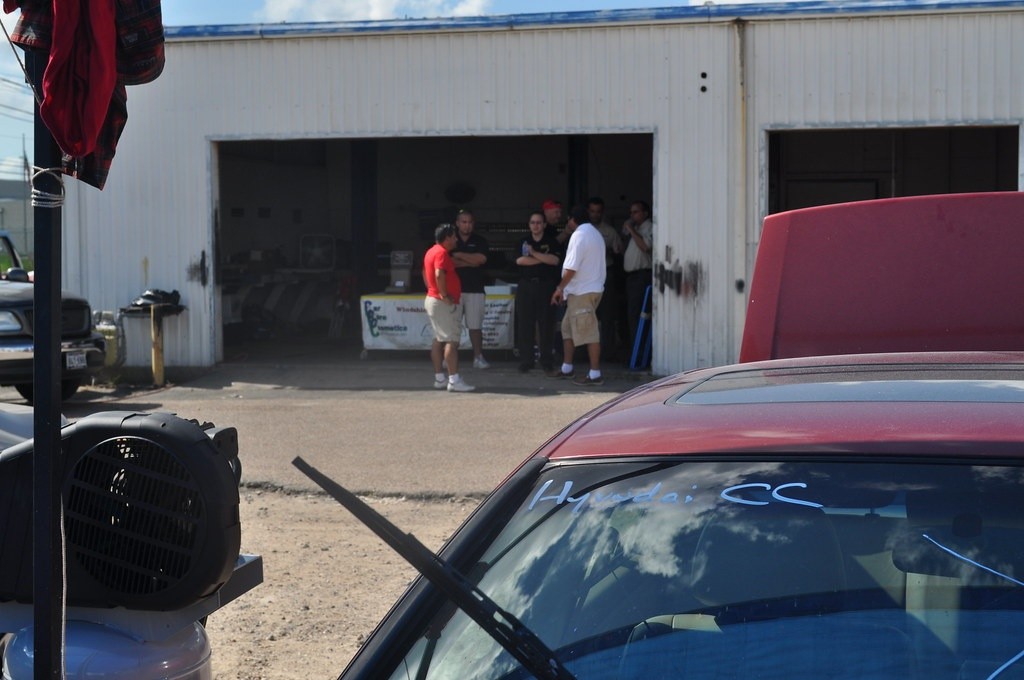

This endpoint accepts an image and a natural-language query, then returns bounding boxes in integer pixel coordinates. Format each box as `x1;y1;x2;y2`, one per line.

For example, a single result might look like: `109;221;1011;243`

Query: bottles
522;241;528;256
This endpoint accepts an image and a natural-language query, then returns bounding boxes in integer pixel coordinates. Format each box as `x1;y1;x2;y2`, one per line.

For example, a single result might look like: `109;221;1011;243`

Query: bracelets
556;286;563;292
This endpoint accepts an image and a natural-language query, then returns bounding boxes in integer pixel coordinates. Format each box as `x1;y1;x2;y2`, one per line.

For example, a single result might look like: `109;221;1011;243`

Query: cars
0;227;105;406
334;187;1024;680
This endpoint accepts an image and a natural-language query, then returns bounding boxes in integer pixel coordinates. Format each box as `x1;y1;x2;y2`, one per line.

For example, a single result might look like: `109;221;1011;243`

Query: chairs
798;459;905;629
618;503;915;680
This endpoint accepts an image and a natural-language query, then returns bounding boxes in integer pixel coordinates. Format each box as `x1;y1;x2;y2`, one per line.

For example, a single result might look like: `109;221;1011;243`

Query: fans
300;233;337;272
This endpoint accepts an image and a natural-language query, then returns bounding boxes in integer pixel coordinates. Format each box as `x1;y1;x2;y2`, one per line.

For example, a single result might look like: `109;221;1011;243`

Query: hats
544;200;560;211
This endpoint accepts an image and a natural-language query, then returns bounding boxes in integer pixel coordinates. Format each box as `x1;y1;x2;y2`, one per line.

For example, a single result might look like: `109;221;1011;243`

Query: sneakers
434;379;476;392
472;358;489;368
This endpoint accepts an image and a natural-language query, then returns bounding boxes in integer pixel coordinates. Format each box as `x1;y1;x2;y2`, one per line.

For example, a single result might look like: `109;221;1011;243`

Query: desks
360;292;514;360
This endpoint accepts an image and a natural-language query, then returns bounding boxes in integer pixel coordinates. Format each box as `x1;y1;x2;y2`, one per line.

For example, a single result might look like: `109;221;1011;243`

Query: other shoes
572;375;604;386
546;370;575;379
519;363;534;371
544;365;554;372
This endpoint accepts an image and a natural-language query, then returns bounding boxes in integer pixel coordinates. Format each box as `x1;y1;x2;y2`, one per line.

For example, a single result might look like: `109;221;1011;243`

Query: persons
546;210;606;385
541;198;577;253
512;212;560;370
422;223;475;392
587;198;622;345
623;202;652;363
442;210;489;370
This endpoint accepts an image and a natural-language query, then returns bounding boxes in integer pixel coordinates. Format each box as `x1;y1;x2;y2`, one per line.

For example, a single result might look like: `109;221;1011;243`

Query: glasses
530;221;542;225
445;222;450;234
630;210;639;214
